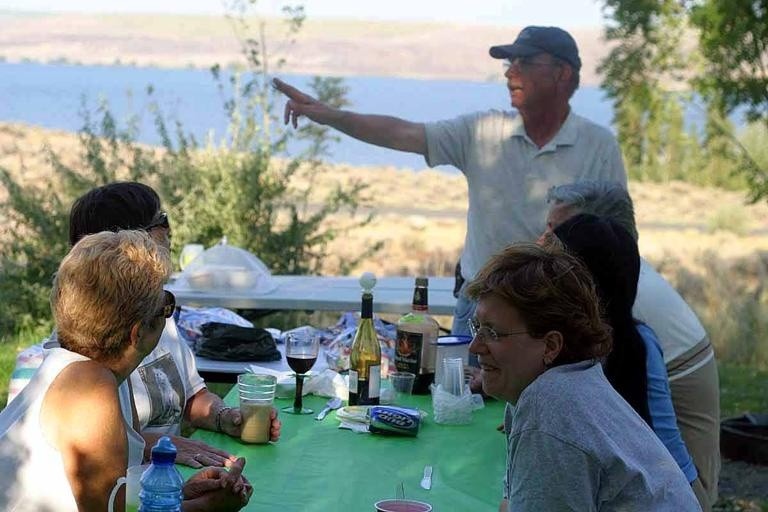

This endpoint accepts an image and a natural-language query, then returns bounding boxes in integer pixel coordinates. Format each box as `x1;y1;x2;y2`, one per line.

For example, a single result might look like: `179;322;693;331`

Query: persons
459;245;703;512
0;228;253;512
7;182;280;469
549;212;711;512
274;27;626;365
536;180;720;512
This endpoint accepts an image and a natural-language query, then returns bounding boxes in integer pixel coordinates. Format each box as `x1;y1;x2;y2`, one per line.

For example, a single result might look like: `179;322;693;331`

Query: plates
334;404;421;425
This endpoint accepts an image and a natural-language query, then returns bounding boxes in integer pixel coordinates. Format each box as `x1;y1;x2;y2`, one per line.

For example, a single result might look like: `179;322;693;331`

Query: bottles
348;292;381;406
395;275;437;395
139;435;185;512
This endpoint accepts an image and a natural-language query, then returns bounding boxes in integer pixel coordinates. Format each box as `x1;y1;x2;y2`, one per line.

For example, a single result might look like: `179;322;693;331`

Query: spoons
317;397;342;420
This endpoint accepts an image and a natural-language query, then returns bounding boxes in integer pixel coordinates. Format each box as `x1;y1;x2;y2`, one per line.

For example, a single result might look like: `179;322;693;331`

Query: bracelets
214;405;231;431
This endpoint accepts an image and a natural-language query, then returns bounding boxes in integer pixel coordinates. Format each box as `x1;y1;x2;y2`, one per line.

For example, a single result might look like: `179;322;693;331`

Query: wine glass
281;331;319;415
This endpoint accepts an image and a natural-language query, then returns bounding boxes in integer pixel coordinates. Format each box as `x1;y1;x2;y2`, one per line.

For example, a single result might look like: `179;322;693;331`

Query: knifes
419;466;434;490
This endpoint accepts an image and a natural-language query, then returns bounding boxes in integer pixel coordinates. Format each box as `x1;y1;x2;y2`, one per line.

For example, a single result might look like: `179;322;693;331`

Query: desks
196;339;327;384
162;275;459;316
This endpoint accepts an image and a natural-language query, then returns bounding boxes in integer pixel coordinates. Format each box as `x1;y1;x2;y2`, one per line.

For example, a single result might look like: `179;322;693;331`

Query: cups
107;465;149;512
390;372;416;406
435;357;466;423
176;243;206;275
233;374;277;446
374;499;433;512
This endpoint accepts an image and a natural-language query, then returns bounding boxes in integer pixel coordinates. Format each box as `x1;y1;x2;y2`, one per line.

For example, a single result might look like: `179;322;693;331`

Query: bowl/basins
186;273;215;291
223;269;260;291
720;417;767;462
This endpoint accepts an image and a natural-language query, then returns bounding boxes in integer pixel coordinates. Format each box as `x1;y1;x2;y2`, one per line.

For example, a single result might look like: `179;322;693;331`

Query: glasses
157;287;177;319
144;211;170;230
465;317;527;346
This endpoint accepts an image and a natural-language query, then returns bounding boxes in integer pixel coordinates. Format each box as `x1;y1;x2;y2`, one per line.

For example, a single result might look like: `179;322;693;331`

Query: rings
193;452;200;459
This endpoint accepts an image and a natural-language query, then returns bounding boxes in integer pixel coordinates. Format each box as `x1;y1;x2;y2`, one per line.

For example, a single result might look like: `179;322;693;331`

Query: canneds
366;406;422;437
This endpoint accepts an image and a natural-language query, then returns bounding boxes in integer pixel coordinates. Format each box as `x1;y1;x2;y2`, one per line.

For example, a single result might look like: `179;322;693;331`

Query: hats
488;24;583;69
